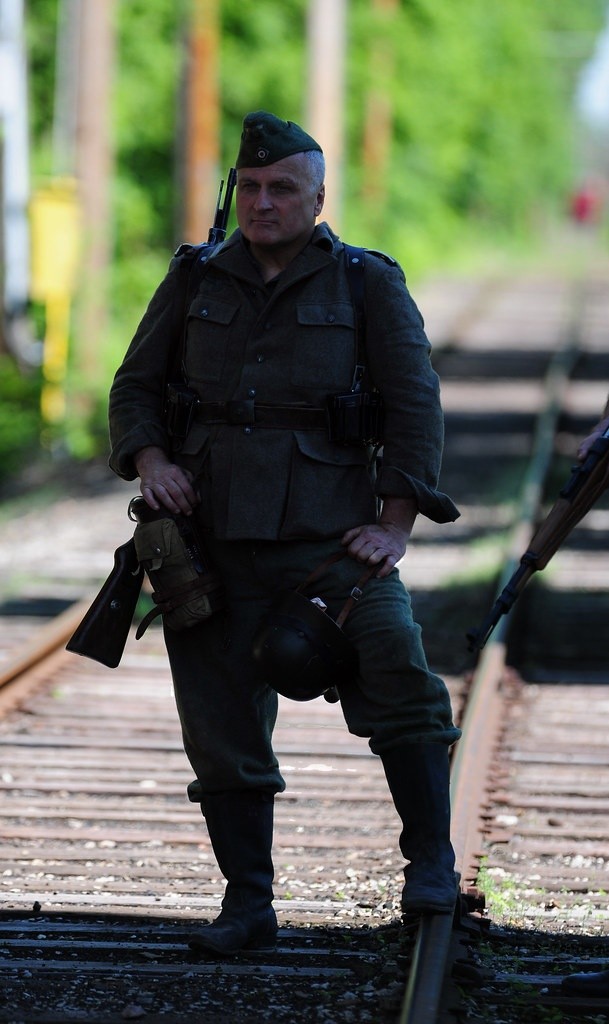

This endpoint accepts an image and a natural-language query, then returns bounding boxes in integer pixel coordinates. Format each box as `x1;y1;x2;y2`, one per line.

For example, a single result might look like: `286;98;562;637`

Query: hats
235;112;323;169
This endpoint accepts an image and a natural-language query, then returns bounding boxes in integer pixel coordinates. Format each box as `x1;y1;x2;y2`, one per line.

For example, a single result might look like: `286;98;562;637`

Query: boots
380;742;460;912
189;790;277;958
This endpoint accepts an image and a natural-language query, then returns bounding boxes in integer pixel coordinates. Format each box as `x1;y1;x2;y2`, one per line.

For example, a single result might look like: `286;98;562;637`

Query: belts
195;399;328;432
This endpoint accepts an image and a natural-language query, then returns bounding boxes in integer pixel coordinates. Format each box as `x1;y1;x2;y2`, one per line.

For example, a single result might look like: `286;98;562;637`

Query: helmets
252;589;357;700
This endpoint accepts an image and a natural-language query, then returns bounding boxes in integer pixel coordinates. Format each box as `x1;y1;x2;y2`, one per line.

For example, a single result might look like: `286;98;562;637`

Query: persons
107;111;461;961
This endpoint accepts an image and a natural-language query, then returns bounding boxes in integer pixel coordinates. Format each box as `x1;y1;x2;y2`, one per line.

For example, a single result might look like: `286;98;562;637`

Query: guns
465;398;609;653
67;166;239;668
